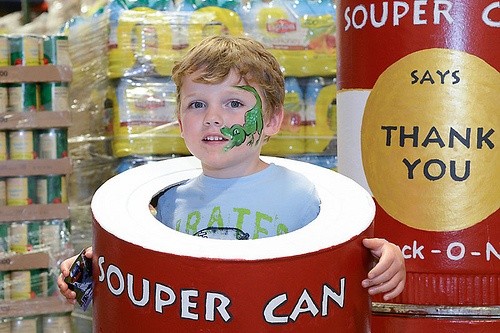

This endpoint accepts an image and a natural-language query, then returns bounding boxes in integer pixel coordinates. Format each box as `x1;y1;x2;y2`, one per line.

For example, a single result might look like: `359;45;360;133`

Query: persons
57;33;408;304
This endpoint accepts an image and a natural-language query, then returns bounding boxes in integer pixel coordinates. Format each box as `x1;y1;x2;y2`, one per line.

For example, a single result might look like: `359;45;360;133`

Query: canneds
0;32;71;333
